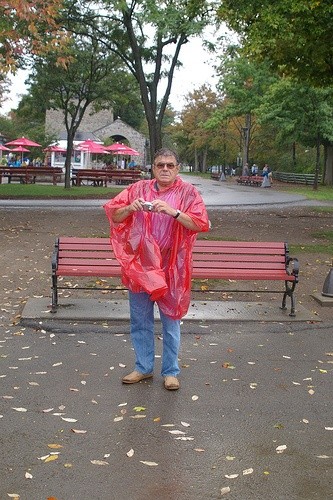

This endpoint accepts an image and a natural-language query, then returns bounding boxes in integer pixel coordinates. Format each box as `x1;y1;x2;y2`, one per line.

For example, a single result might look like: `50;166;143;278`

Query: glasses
153;161;178;169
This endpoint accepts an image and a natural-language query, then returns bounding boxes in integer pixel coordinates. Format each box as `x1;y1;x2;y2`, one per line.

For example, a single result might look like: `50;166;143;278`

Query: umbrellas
73;138;140;165
0;142;11;151
9;145;31;165
43;144;68;167
4;136;43;166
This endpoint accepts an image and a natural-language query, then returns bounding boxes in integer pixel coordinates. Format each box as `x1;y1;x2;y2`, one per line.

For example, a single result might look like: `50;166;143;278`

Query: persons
91;157;148;171
242;161;273;186
5;152;44;167
106;146;210;392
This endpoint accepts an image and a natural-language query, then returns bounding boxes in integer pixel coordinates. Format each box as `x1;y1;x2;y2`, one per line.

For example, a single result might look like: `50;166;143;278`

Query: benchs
0;164;143;186
210;173;265;187
48;233;300;316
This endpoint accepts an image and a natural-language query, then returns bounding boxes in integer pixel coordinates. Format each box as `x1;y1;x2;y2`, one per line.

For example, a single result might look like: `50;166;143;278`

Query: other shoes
164;375;180;389
122;370;153;383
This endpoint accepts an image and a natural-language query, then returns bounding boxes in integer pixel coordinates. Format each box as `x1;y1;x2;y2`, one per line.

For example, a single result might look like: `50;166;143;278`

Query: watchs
173;208;180;218
